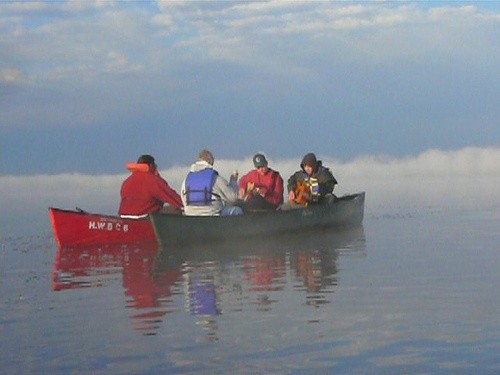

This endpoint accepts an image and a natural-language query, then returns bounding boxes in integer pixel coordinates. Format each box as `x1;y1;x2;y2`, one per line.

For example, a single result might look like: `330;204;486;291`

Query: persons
287;152;338;208
232;153;284;213
180;149;245;216
119;154;183;219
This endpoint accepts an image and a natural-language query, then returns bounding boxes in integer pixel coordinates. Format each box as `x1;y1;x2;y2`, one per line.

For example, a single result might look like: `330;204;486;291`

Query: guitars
289;179;311;205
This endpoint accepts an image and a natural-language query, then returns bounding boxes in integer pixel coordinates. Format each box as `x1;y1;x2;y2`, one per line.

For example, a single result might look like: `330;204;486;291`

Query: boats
149;190;367;247
51;243;159;294
47;205;156;247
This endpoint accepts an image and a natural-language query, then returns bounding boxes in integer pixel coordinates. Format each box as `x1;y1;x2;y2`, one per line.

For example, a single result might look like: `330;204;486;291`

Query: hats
253;153;268;167
